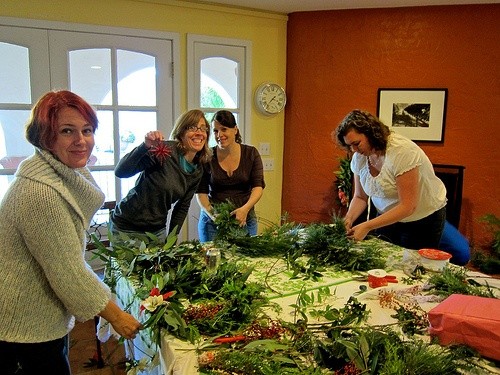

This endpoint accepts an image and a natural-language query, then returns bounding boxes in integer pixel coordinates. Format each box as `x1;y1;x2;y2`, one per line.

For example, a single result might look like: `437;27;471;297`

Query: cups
368;268;388;288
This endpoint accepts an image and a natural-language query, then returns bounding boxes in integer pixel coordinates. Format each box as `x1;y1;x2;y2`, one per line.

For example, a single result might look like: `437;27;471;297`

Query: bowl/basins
418;249;452;270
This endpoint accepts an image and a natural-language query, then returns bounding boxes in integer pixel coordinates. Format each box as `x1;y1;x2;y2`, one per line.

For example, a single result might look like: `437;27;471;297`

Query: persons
335;108;447;248
195;110;266;243
110;110;212;246
0;90;143;375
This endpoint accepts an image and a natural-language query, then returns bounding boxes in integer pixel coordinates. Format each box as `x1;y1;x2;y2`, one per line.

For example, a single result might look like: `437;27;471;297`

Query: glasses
185;125;209;132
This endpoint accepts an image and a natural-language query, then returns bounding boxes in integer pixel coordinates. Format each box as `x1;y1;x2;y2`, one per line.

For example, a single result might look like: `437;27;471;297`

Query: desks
96;223;500;375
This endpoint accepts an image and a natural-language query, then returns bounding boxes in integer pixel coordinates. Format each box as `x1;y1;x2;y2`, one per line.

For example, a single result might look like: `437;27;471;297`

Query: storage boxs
428;294;500;361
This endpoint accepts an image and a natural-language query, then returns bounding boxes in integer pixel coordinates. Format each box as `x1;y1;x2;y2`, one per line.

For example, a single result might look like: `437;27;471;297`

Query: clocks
255;82;287;116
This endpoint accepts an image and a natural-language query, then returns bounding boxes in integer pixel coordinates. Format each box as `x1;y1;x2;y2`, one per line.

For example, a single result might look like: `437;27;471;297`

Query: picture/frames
377;88;447;144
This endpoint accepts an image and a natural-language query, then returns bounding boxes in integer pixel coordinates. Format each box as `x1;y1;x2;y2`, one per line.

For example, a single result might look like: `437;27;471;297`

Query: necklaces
369;152;381;166
